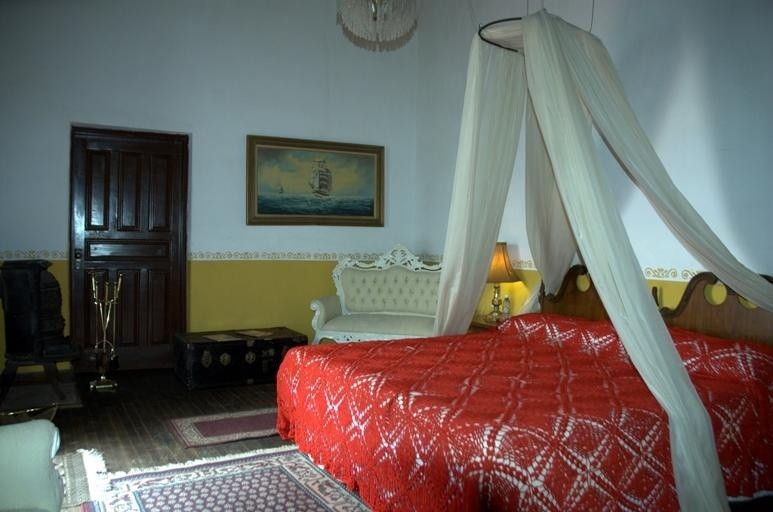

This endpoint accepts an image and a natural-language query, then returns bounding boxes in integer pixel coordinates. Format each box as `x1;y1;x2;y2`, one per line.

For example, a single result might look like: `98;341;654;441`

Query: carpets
58;449;108;508
81;444;373;512
167;406;278;449
0;380;83;409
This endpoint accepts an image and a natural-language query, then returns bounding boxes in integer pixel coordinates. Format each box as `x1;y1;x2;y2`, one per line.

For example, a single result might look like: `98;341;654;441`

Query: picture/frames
244;134;384;229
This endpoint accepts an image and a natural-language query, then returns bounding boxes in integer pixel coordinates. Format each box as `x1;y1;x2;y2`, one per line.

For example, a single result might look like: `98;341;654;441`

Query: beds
274;263;773;512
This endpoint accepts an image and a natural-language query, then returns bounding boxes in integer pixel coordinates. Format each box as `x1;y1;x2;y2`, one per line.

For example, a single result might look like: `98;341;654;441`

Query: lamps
334;0;420;56
483;241;520;323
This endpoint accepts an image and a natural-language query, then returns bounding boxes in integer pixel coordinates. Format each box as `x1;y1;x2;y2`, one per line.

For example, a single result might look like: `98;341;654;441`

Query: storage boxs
171;327;307;392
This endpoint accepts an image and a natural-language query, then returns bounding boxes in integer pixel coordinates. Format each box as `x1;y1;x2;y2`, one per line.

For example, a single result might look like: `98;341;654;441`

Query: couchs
308;243;443;345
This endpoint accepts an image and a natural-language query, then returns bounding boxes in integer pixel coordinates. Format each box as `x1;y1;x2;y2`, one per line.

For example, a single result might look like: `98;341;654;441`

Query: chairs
0;259;87;400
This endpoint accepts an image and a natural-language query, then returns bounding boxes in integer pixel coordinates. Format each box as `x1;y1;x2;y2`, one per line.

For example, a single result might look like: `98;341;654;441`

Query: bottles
501;293;511;313
